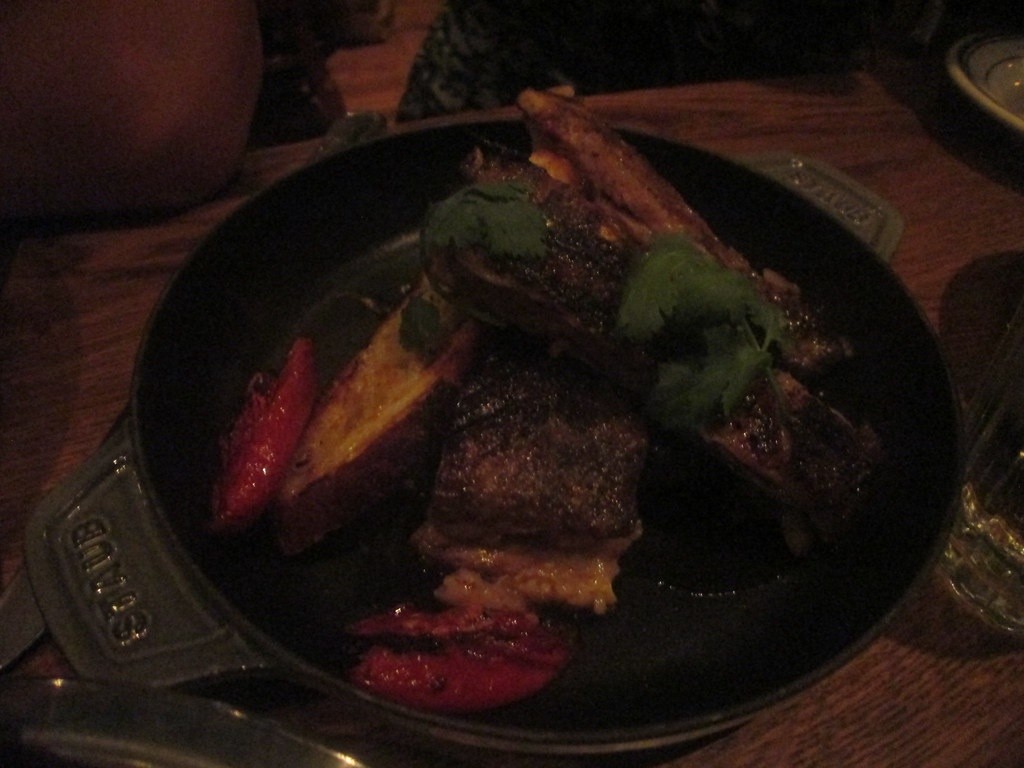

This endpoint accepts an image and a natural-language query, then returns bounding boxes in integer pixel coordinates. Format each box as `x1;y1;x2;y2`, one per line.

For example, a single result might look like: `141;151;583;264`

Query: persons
393;0;1024;125
0;0;267;218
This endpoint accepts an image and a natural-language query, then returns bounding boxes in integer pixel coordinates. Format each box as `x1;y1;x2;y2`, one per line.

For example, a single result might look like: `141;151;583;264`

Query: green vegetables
395;182;792;432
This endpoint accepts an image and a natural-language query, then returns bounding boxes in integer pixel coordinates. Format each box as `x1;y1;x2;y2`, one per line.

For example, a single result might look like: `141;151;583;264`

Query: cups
935;300;1024;638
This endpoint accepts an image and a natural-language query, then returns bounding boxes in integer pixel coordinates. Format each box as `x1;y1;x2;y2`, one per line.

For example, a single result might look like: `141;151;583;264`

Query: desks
0;46;1024;768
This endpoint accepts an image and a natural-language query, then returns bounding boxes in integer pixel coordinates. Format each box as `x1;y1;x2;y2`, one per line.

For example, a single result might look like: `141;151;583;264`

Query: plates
944;27;1024;136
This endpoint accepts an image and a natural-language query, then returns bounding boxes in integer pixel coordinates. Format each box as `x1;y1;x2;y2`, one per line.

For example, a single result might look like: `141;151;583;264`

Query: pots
23;115;968;768
